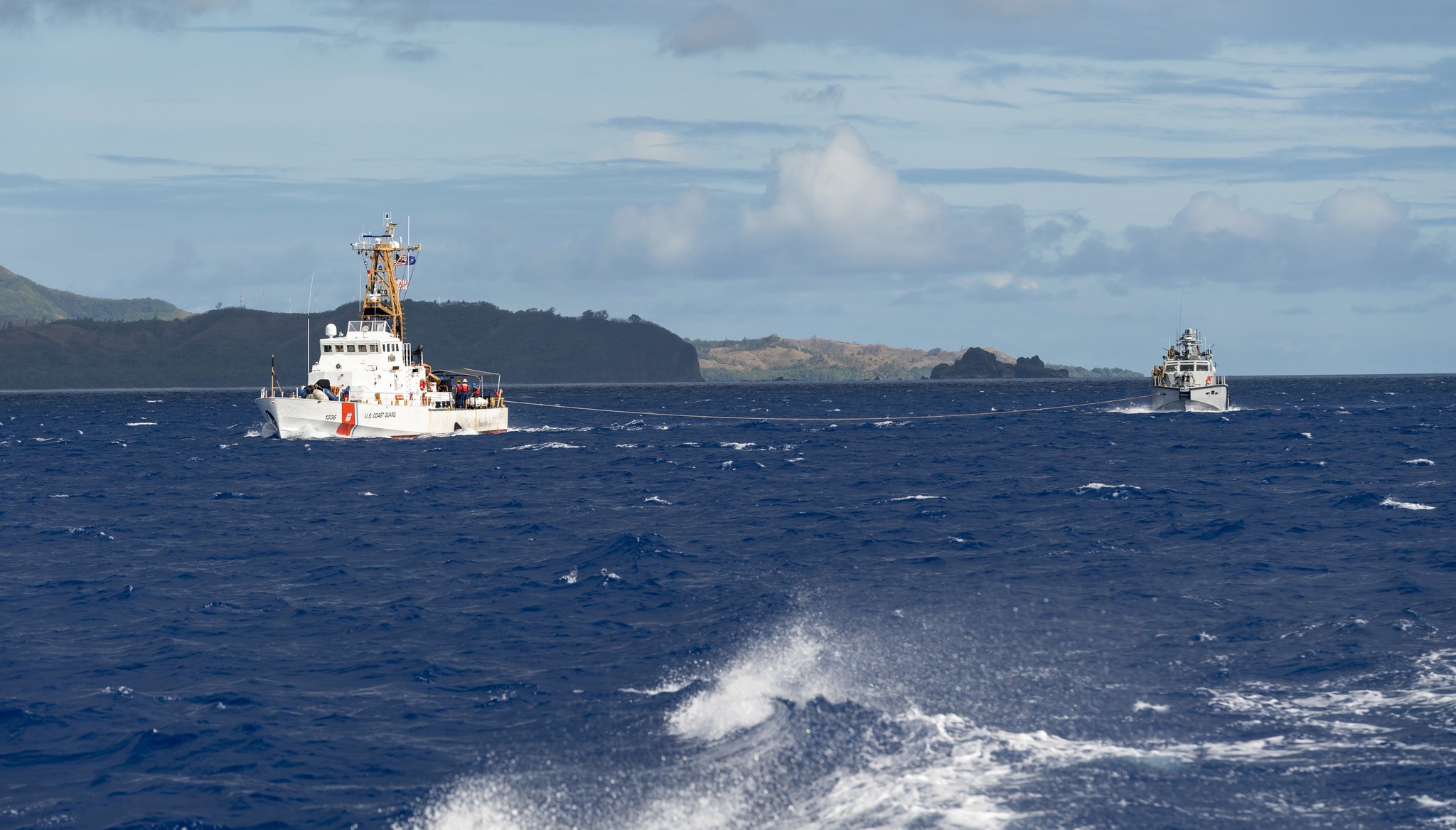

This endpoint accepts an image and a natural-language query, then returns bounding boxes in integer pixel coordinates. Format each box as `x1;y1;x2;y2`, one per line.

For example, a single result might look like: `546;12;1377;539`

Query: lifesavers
1206;376;1211;384
420;379;425;391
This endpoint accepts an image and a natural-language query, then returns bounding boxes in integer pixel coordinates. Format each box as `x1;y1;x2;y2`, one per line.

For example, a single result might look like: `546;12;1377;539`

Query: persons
1168;346;1180;360
1196;345;1205;356
436;376;445;409
460;379;470;407
363;317;372;332
470;383;480;398
446;379;454;407
1151;364;1165;386
456;379;462;408
381;316;393;334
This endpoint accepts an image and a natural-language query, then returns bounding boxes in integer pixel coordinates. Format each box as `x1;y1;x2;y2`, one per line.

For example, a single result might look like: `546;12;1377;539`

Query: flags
394;254;416;265
394;276;408;290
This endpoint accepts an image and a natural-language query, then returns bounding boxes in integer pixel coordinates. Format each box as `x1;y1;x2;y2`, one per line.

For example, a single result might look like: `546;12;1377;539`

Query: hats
387;316;391;319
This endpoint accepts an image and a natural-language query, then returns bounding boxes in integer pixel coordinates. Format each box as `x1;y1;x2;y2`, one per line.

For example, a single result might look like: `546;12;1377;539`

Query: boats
254;209;508;442
1148;276;1231;415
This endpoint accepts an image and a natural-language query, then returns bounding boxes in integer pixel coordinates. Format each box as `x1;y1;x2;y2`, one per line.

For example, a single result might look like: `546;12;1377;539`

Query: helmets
440;377;444;380
471;383;476;386
456;379;461;382
463;379;468;382
